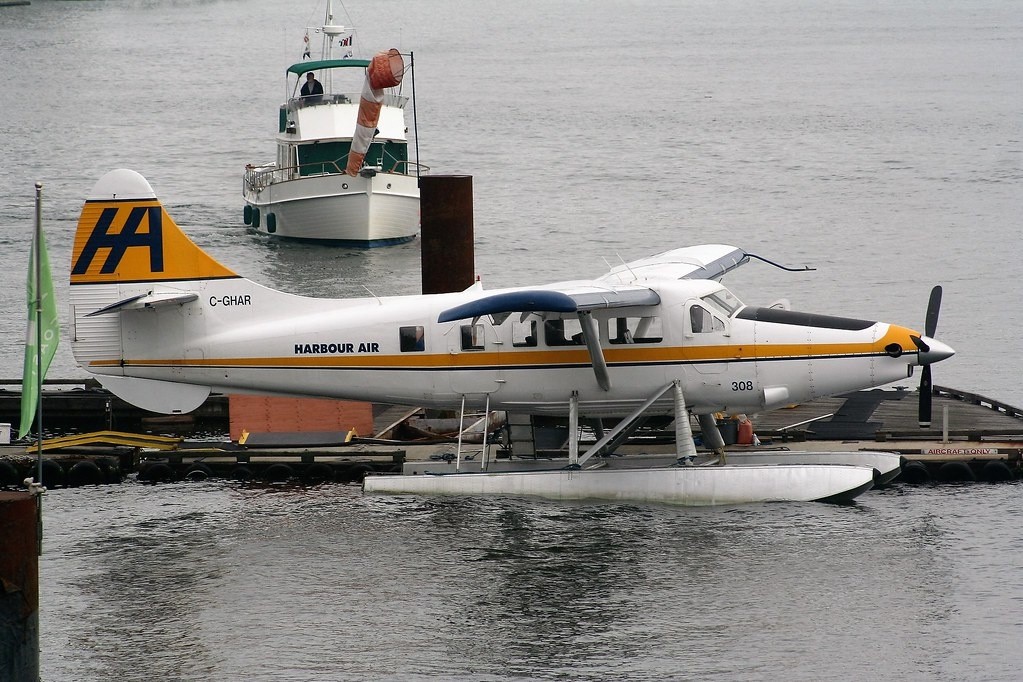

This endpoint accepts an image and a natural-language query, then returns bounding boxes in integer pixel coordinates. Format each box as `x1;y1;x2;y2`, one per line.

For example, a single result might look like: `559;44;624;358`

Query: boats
237;0;429;250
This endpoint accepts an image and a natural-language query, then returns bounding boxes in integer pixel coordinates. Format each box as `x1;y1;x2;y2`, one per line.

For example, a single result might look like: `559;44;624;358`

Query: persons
301;72;323;104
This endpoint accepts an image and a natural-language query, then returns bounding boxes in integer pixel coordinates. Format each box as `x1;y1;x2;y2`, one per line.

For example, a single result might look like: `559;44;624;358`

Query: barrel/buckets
0;423;11;444
716;419;737;444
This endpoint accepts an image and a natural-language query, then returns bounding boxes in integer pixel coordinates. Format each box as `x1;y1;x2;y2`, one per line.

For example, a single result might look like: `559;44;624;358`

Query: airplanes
61;165;960;505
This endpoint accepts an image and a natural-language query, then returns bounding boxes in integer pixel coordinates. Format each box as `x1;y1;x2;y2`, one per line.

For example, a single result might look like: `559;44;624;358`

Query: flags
18;225;59;440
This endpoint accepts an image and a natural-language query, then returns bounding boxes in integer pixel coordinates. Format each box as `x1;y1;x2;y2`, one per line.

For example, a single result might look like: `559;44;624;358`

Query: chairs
524;327;636;344
